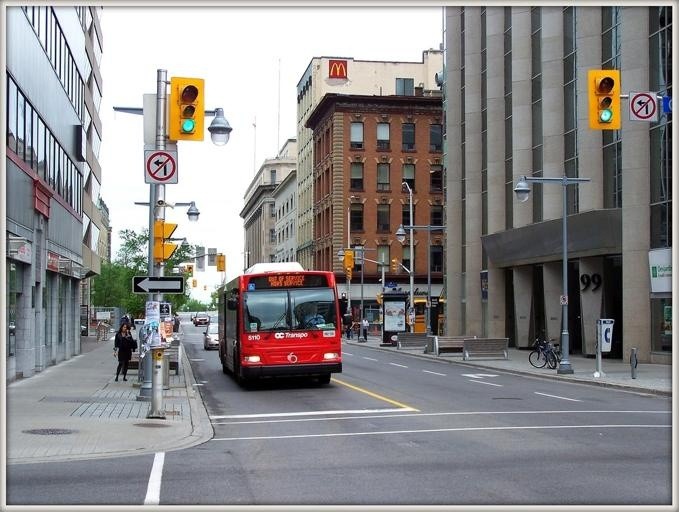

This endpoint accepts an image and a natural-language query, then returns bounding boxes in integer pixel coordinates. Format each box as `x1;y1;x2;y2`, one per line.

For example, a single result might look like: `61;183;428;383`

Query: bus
217;262;343;391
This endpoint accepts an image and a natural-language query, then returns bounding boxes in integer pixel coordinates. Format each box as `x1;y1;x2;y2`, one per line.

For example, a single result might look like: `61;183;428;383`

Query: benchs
127;347;178;374
397;332;508;360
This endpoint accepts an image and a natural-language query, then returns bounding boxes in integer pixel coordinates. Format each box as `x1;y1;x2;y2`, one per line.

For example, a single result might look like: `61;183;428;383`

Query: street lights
514;174;592;374
133;200;202;375
112;66;232;380
336;195;386;343
395;223;447;353
401;181;416;332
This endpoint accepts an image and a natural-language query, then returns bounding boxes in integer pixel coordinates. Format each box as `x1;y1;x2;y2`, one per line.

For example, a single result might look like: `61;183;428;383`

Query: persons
363;318;369;341
113;315;137;382
346;318;359;339
305;304;325;328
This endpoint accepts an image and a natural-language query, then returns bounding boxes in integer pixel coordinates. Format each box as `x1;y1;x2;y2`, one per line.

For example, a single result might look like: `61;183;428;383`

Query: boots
115;377;128;381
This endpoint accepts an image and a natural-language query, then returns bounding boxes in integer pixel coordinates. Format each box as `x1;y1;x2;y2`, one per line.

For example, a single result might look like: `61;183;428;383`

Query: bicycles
528;333;562;369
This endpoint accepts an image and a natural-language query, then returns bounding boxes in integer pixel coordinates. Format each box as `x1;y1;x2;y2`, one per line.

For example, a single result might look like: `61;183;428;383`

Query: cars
190;312;219;351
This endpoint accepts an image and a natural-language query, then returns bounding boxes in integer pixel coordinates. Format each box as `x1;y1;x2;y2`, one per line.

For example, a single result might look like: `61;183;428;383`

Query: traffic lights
170;76;204;142
587;69;622;129
187;265;193;277
